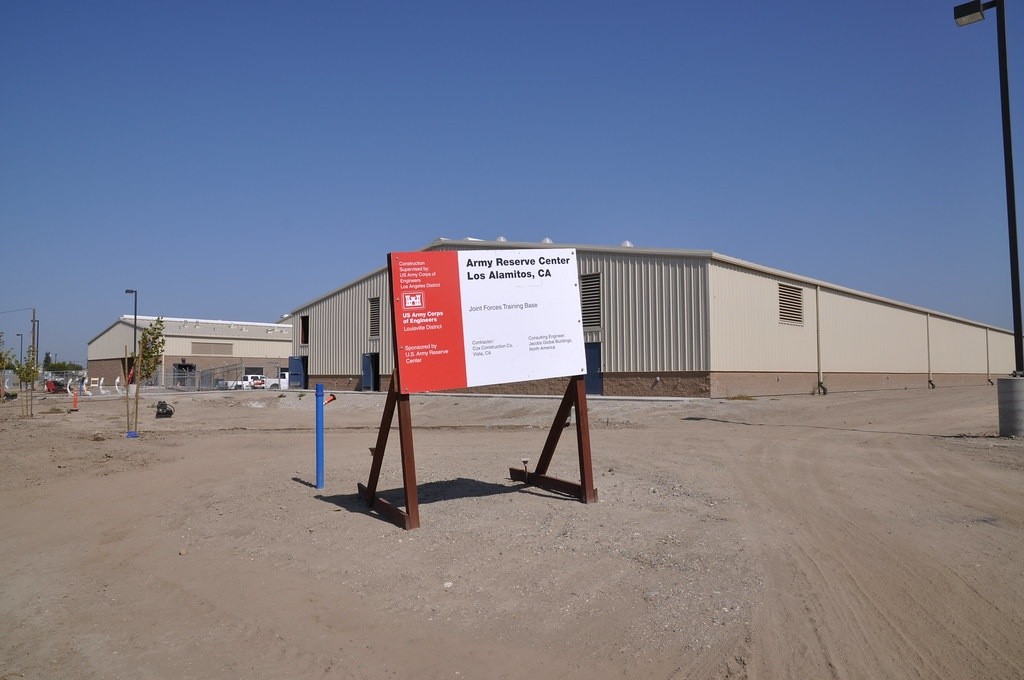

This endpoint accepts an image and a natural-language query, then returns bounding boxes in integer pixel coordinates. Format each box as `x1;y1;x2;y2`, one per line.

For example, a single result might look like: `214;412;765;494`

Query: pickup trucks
218;374;266;390
252;371;289;390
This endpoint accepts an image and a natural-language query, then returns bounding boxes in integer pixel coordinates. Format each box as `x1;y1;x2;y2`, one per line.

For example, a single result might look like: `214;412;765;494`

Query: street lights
55;353;58;364
16;333;23;366
31;319;39;372
126;289;139;387
952;1;1024;453
